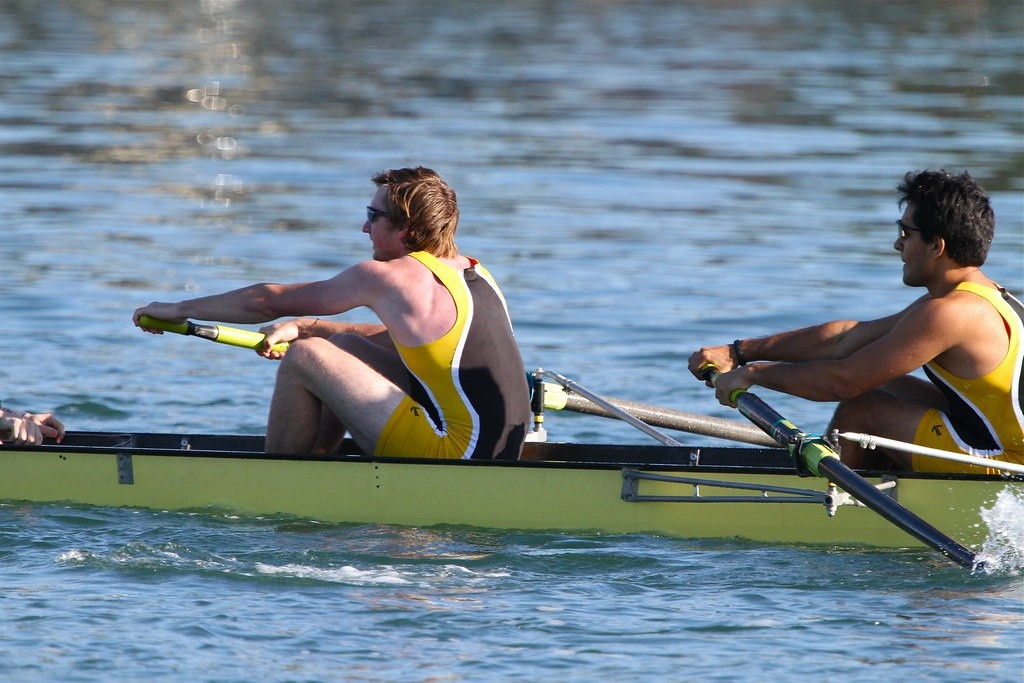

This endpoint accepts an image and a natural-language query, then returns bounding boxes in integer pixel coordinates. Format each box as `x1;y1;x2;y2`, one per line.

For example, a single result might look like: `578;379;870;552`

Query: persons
688;175;1024;475
0;406;65;445
132;166;531;460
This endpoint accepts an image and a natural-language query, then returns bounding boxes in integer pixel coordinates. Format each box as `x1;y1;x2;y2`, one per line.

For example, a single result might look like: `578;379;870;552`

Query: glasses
367;206;397;222
896;219;926;239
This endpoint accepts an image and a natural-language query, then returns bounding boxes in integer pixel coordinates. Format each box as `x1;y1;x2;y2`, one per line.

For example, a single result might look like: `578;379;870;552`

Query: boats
1;367;1024;553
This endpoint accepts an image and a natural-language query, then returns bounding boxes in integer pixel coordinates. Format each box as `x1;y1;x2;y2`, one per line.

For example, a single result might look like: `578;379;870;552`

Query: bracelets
734;340;746;366
